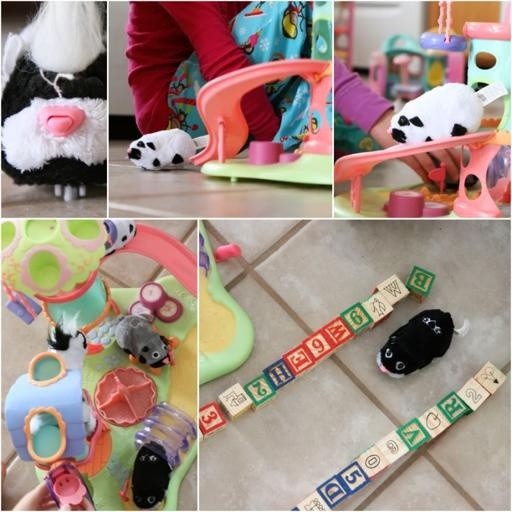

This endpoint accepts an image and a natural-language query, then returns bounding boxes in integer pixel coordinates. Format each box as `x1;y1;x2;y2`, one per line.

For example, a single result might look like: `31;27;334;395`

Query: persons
2;462;60;510
126;1;333;155
334;52;400;148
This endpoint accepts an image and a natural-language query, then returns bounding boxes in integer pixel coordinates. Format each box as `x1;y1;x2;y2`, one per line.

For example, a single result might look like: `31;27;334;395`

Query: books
376;308;471;379
